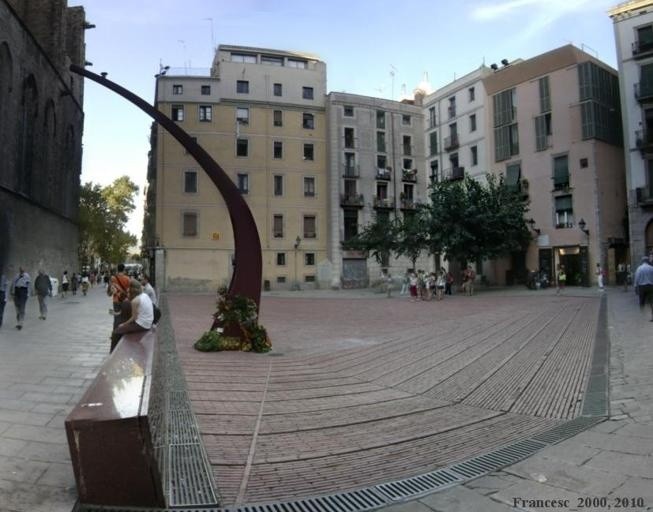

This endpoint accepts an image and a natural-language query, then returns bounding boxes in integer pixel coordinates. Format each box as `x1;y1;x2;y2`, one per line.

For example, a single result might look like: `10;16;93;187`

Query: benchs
62;330;167;508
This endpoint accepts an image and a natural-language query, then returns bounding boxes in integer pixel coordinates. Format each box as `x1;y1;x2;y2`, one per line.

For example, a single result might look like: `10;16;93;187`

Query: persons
0;264;157;354
387;256;653;310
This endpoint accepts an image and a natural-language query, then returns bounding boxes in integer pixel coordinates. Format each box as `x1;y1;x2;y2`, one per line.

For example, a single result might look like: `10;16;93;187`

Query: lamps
578;216;589;237
527;216;540;235
